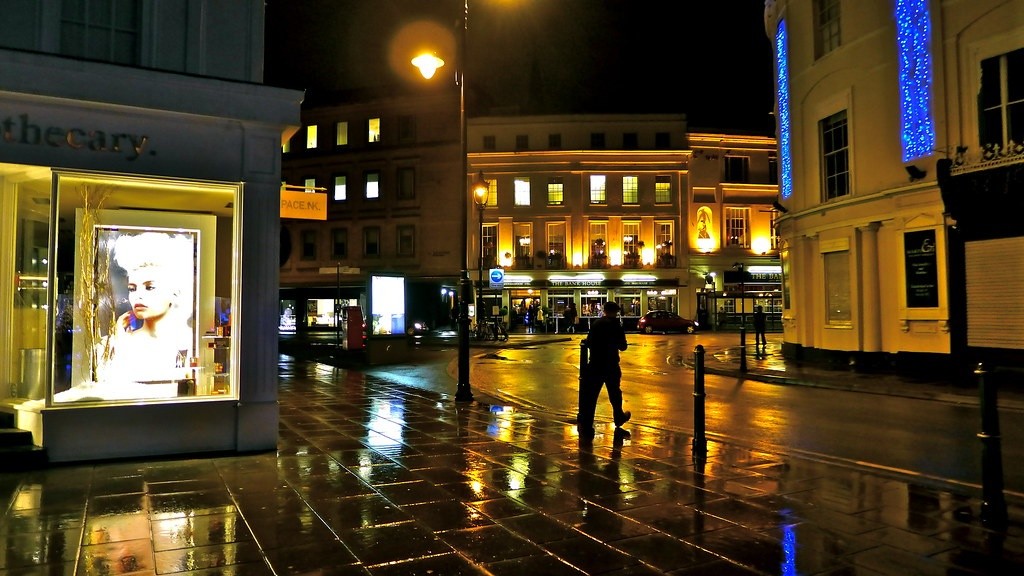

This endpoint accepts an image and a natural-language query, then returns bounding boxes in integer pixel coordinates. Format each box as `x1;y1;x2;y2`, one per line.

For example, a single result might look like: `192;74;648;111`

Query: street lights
409;28;474;403
710;270;720;331
472;170;491;321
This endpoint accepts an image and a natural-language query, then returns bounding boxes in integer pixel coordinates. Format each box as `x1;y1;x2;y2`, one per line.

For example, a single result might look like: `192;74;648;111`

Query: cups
189;358;197;367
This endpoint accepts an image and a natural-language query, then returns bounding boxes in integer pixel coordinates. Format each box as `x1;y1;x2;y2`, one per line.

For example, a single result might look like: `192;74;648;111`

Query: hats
604;301;621;312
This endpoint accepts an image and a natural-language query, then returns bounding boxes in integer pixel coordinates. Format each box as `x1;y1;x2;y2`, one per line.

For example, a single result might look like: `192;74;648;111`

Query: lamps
773;202;788;214
505;253;512;259
762;252;766;256
706;252;711;256
905;165;927;182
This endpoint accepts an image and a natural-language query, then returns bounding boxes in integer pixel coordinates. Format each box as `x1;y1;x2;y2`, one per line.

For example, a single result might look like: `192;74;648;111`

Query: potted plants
500;307;508;333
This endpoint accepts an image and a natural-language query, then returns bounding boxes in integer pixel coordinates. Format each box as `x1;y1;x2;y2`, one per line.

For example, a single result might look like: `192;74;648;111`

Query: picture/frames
71;206;218;400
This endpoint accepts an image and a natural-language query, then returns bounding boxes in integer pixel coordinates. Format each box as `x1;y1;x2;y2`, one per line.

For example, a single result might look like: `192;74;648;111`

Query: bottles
175;351;185;368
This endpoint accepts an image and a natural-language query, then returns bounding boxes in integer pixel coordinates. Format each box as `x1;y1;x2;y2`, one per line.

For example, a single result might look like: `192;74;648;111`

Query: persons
564;301;577;334
753;306;767;350
97;232;195;379
528;305;535;333
718;307;727;325
582;302;632;427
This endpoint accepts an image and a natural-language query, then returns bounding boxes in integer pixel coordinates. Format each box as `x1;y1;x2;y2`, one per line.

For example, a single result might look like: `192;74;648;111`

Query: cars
637;310;700;334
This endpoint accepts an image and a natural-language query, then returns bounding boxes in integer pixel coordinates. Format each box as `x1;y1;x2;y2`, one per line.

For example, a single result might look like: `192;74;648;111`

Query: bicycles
470;317;511;341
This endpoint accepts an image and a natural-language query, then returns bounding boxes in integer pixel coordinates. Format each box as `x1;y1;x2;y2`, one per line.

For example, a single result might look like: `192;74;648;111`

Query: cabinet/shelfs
202;334;233;396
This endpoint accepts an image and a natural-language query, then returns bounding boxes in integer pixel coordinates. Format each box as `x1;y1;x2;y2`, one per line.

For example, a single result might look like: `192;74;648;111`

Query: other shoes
613;411;631;426
